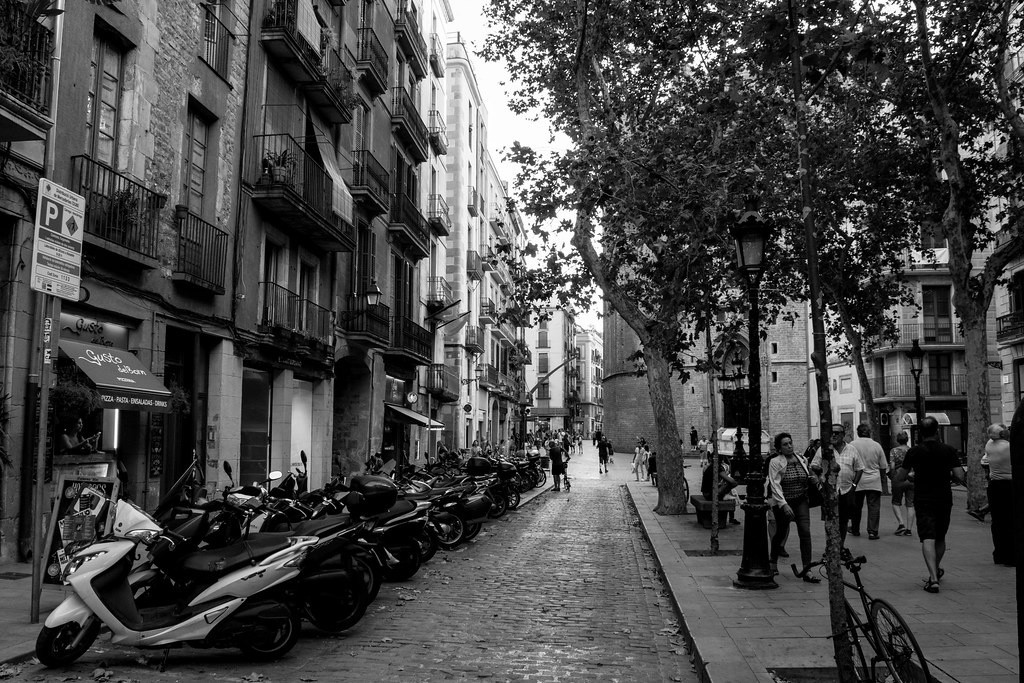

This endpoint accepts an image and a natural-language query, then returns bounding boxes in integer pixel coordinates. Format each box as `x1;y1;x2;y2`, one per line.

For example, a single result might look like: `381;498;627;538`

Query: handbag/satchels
806;483;825;508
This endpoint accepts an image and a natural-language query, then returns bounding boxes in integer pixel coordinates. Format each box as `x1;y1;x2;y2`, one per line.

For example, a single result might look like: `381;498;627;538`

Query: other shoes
779;546;789;558
729;518;741;525
904;530;912;536
968;508;984;521
770;563;779;575
895;524;905;533
803;574;821;582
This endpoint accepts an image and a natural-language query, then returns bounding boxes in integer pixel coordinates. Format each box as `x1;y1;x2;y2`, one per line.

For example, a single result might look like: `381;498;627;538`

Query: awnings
901;412;961;431
384;404;445;431
58;339;172;413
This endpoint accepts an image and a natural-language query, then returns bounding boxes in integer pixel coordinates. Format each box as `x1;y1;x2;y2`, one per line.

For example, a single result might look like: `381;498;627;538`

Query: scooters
155;439;539;581
35;488;319;666
130;503;372;634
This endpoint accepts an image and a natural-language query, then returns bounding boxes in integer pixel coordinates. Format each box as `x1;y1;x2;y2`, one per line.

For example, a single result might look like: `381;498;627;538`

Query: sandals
847;526;861;537
868;531;880;540
924;568;944;593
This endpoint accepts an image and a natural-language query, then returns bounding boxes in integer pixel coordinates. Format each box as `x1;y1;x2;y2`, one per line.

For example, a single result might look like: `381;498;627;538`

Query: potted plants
168;380;191;415
254;320;335;354
49;381;103;415
265;148;300;182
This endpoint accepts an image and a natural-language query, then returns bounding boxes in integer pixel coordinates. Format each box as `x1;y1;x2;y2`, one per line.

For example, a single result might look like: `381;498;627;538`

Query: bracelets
852;483;857;487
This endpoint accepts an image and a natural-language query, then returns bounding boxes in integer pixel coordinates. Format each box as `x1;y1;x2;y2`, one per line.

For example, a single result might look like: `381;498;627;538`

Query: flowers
89;183;153;228
152;172;171;194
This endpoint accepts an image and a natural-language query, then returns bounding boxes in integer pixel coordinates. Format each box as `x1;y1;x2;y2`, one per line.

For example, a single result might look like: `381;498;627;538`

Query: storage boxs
467;457;491;475
527;449;540;462
350;475;397;517
497;462;517;481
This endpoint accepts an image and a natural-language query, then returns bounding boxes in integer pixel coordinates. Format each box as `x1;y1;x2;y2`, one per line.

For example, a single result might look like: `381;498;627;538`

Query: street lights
904;338;926;444
729;353;747;458
730;210;776;584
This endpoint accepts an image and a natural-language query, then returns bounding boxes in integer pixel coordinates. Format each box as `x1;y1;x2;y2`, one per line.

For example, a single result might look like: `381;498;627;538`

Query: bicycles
563;457;572;492
510;452;546;488
791;542;931;683
684;464;690;501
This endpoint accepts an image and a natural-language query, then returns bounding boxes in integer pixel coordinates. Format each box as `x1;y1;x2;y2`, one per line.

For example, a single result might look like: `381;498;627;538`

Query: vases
174;205;189;219
105;226;125;243
147;194;168;208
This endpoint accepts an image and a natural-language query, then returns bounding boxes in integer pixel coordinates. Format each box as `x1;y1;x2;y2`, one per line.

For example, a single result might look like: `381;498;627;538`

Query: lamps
340;277;383;325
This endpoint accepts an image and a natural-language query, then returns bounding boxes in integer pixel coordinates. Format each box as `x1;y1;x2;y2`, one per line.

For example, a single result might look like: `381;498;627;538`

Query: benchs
691;496;736;529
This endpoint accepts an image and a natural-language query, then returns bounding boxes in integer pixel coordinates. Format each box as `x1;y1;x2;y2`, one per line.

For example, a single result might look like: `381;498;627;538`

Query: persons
528;429;583;457
980;422;1015;567
897;416;965;593
437;441;448;461
762;451;789;558
471;436;491;457
803;438;821;464
633;437;656;481
593;429;614;474
61;415;102;452
690;426;741;525
847;423;889;540
889;432;914;535
768;433;822;584
548;441;571;491
810;424;865;548
